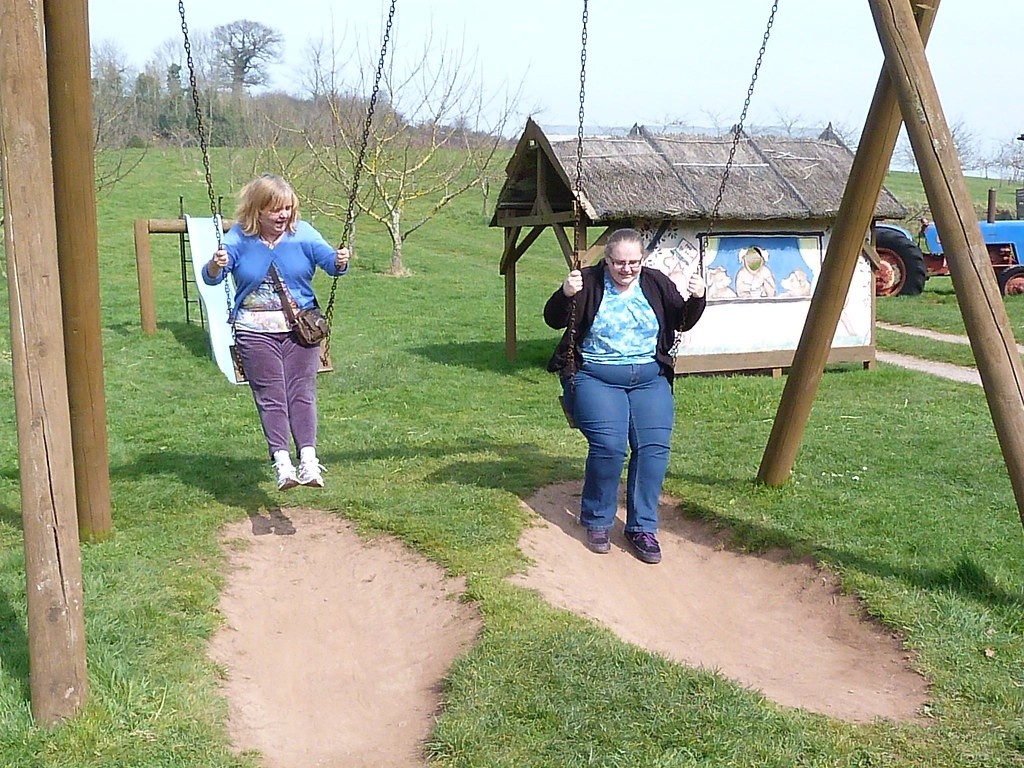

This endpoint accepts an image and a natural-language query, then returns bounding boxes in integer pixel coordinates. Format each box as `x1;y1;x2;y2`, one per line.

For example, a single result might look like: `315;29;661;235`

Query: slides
181;214;249;387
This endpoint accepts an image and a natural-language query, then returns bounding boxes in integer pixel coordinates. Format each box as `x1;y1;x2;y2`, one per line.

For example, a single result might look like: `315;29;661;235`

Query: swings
168;2;399;386
559;0;781;429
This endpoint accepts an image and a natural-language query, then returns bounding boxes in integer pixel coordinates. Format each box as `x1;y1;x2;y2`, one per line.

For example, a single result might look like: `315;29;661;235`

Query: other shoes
624;524;662;563
588;528;611;553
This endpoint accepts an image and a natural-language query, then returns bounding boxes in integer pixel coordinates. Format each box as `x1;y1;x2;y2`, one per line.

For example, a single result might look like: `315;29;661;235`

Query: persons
544;228;707;565
202;174;350;490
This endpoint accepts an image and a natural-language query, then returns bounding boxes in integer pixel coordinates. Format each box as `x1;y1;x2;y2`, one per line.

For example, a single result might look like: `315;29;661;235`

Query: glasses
608;255;643;268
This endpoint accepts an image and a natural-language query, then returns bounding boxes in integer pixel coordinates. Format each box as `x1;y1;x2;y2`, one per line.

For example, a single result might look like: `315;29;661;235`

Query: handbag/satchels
291;307;329;347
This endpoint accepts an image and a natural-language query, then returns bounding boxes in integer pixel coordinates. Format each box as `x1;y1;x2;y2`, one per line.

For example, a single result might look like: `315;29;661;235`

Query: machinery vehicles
872;187;1024;302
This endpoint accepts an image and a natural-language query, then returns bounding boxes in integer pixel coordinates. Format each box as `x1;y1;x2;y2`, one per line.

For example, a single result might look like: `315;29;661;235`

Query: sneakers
299;457;327;487
272;462;301;491
